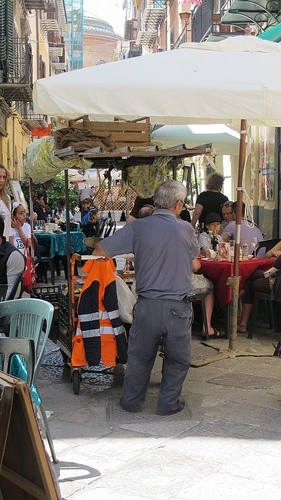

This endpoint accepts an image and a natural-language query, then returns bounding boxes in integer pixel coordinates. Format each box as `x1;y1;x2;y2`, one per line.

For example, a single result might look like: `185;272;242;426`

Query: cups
116;258;126;269
206;249;213;260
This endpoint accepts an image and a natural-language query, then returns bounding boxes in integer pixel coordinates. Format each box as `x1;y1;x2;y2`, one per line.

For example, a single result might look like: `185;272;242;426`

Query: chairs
0;298;58;464
190;239;281;340
96;217;116;239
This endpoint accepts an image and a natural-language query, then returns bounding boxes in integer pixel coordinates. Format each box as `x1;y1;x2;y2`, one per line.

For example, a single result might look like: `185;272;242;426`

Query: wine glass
250;237;260;260
34;220;44;230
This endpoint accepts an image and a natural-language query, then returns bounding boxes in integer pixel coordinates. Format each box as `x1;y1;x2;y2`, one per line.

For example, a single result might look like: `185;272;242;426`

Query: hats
205;213;222;225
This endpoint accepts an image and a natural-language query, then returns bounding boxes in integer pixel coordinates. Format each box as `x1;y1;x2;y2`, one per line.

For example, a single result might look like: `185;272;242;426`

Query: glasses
177;199;187;210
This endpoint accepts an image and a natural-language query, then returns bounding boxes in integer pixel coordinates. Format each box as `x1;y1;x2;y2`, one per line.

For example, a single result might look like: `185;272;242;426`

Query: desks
34;232;84;285
197;255;272;305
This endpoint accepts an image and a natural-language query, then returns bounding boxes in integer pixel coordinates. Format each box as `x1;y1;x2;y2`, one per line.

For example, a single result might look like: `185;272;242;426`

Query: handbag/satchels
21;257;38;295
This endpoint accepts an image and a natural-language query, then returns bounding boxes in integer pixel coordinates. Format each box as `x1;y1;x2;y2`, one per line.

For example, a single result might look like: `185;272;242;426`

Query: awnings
220;0;281;34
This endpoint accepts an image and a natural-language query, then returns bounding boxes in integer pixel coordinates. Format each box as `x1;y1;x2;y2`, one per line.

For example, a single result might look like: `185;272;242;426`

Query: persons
237;241;281;332
179;201;264;260
189;173;228;229
80;198;98;238
52;198;74;223
138;204;226;339
33;193;52;223
90;180;200;414
0;164;37;302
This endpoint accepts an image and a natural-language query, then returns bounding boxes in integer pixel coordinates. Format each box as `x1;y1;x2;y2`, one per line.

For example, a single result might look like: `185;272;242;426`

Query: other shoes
155;398;185;415
119;396;144;412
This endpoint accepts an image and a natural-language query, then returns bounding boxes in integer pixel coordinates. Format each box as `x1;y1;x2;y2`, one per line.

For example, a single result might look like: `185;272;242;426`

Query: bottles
45;214;58;234
211;230;248;262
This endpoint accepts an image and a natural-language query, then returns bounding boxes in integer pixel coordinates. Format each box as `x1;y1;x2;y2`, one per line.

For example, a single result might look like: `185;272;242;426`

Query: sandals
202;328;226;338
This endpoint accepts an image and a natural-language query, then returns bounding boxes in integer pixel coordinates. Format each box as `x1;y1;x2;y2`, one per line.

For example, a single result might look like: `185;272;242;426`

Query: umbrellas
31;35;281;127
150;124;247;156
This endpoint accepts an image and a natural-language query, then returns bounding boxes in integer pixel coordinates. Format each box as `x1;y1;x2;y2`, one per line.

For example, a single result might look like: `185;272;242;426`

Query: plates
33;230;44;233
